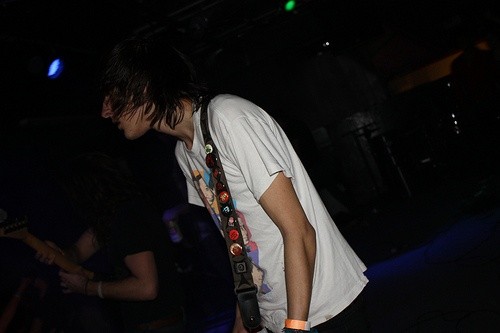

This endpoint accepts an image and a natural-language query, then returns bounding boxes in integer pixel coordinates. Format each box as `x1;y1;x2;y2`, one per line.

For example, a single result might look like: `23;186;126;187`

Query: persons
295;38;500;218
35;167;185;333
101;30;377;333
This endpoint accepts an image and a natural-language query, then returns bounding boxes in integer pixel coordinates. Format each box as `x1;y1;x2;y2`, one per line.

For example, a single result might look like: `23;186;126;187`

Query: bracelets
97;281;107;299
81;278;89;297
281;318;311;333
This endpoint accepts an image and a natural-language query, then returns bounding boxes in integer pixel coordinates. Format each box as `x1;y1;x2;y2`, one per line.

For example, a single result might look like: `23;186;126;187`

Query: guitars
0;213;95;281
